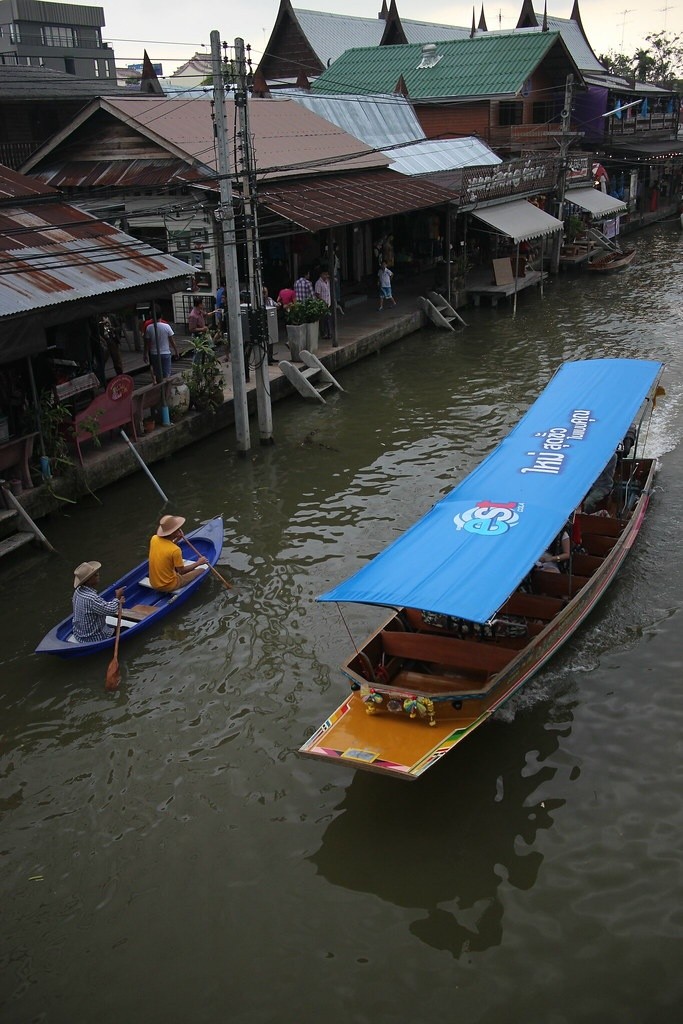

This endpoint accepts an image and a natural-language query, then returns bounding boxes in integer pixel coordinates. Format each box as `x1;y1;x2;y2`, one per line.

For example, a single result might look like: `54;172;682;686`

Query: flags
641;99;647;117
615;100;621;119
666;99;672;112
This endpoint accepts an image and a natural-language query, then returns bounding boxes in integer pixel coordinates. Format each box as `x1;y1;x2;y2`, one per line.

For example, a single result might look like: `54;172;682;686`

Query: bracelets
557;556;560;562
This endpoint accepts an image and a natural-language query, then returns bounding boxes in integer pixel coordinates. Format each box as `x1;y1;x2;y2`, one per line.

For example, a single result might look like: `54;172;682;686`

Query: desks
561;241;597;257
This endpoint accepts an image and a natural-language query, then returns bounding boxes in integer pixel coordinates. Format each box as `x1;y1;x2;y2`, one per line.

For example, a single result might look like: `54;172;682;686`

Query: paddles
181;535;233;590
105;601;123;690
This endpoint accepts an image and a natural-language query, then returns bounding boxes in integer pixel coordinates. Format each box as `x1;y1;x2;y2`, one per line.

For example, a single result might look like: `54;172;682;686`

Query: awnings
564;186;627;262
471;199;564;314
611;140;683;220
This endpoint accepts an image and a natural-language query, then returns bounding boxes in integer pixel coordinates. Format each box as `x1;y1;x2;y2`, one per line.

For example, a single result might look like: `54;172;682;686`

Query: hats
157;515;186;537
74;560;102;588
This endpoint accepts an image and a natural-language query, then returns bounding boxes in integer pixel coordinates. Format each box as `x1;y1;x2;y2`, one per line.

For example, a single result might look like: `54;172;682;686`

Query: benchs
129;376;175;438
139;577;184;593
0;431;38;490
73;374;137;467
380;490;636;679
105;608;145;630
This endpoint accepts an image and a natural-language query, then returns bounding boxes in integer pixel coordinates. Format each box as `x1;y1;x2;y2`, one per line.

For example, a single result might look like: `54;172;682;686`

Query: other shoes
391;303;397;307
377;307;384;311
270;359;279;363
322;335;331;338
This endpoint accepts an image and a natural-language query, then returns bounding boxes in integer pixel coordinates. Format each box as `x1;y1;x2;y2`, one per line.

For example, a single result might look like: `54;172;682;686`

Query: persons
72;562;129;643
213;284;226;323
543;525;570;572
143;303;179;385
104;312;122;376
378;260;396;311
149;515;209;592
189;298;221;366
584;452;617;514
315;269;332;339
293;269;317;303
262;281;296;362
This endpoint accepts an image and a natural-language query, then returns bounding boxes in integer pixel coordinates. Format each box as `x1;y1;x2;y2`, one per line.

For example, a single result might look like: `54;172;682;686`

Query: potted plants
180;332;233;416
280;296;330;363
23;391;74;480
449;251;475;292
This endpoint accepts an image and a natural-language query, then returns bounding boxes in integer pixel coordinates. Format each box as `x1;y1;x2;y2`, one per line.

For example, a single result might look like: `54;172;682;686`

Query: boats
31;509;224;665
581;249;638;272
297;358;663;781
